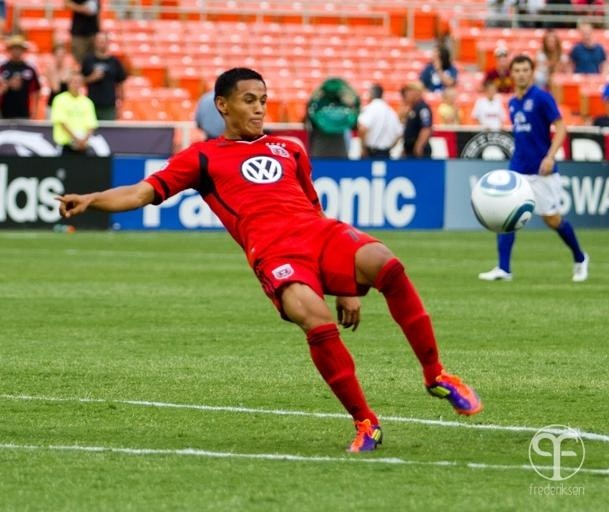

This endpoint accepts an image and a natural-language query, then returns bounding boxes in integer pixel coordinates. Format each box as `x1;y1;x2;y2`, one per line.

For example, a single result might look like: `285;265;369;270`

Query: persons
56;66;482;455
0;1;608;160
477;55;589;284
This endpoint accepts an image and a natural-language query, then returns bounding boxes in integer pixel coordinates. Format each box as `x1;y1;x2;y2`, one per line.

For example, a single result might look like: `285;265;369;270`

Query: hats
6;36;28;52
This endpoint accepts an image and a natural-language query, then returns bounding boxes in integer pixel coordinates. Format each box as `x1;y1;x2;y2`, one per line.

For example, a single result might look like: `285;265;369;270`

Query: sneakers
572;252;589;282
479;266;514;281
348;419;382;452
425;368;484;417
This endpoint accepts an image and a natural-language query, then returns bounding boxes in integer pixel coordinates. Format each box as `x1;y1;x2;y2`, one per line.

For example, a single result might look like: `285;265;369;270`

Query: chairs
0;0;608;127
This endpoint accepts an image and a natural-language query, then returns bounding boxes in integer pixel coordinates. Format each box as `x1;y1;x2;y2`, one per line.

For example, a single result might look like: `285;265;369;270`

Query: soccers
471;170;535;233
